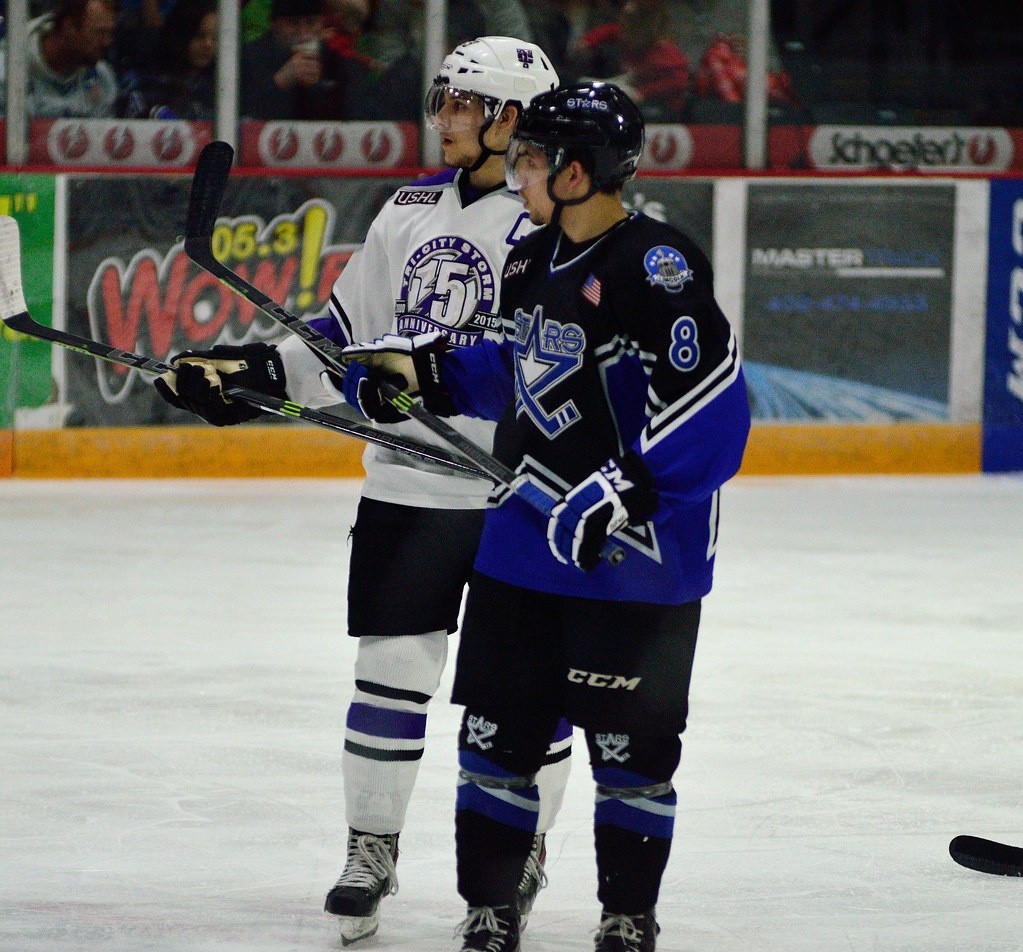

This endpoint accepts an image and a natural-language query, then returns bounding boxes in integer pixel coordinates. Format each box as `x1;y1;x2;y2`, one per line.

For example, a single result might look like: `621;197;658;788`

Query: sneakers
452;904;520;952
514;833;547;931
323;828;402;948
588;909;660;952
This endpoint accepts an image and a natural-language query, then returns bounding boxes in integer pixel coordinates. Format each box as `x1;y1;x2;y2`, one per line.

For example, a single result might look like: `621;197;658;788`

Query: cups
289;37;321;85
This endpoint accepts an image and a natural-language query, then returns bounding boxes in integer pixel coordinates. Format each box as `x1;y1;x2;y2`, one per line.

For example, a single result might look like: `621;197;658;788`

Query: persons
154;35;560;944
0;0;816;127
320;80;752;952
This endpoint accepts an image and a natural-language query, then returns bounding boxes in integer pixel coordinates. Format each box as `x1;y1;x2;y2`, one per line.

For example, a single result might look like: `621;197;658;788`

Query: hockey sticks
181;139;628;565
948;835;1023;877
0;212;497;486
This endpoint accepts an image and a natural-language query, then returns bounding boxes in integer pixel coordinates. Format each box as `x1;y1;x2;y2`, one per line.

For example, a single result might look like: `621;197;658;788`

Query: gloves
155;342;293;427
344;330;444;355
546;450;660;575
326;338;464;425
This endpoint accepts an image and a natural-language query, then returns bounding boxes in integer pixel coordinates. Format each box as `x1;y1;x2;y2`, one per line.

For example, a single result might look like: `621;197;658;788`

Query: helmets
432;36;559;122
514;80;645;187
